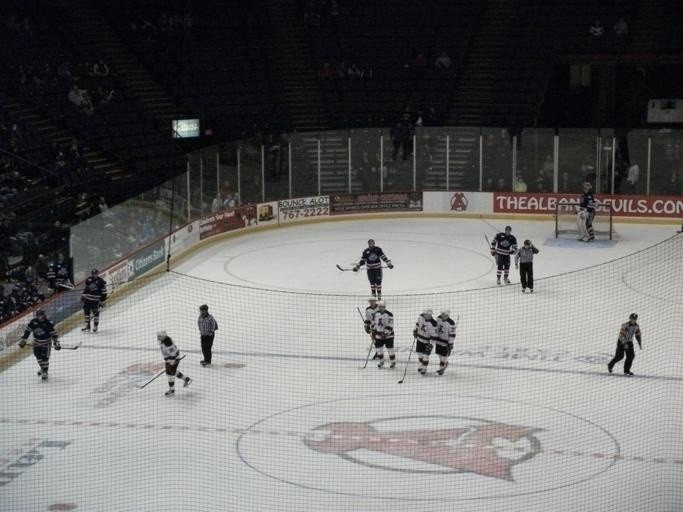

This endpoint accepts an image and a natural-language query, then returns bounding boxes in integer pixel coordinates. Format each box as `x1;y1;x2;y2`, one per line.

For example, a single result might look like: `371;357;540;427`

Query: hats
368;296;450;318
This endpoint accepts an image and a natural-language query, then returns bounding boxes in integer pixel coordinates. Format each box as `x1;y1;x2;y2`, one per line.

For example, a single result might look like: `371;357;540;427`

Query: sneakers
608;363;613;372
624;371;633;375
82;325;90;331
93;326;98;331
37;369;48;379
497;278;533;294
378;361;449;375
164;361;211;395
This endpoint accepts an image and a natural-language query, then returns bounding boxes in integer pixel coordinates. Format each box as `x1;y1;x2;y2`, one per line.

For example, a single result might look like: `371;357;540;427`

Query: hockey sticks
398;334;417;383
25;341;82;350
438;315;459;372
337;265;390;271
133;355;184;389
356;332;374;369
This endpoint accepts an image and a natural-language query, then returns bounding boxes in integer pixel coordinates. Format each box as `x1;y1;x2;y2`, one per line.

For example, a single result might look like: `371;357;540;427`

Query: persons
413;52;451;71
608;313;643;375
80;268;107;332
354;106;439;190
484;133;680;196
363;298;456;374
319;60;374;81
211;121;289;213
491;226;539;293
19;310;60;380
156;331;191;396
304;2;339;27
579;183;596;242
590;18;629;37
0;2;195;321
197;305;216;366
354;239;394;302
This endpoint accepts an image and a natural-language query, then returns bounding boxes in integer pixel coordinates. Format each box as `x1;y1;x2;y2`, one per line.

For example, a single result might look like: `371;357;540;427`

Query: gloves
509;250;514;255
81;294;86;302
100;301;106;307
53;341;61;351
491;252;496;256
353;267;360;272
388;263;393;269
18;340;27;348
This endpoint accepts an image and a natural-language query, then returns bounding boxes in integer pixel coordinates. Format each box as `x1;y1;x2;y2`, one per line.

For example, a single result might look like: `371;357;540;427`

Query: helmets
524;240;531;245
36;309;45;317
199;305;209;310
505;226;512;231
92;269;98;274
629;313;638;320
368;239;375;244
156;330;167;336
